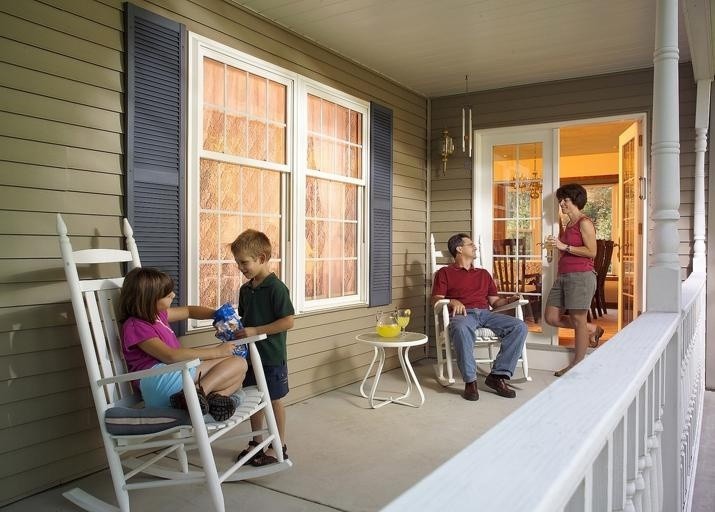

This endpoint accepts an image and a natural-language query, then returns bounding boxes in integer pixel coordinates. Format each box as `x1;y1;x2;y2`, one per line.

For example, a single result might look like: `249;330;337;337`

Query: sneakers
206;392;236;421
170;371;209;415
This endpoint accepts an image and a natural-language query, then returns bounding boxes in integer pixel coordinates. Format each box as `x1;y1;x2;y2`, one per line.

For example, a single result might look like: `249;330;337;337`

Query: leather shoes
485;374;516;398
464;382;479;401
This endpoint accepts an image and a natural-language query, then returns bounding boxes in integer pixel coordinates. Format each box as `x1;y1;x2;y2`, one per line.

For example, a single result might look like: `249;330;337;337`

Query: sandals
238;441;263;466
589;326;604;348
555;369;566;377
252;444;288;467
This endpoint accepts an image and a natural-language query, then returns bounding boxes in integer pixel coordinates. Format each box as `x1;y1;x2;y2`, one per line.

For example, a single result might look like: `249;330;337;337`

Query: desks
356;332;428;411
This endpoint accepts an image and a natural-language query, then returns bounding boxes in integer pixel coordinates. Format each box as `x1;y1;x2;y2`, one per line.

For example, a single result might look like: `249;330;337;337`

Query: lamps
440;128;454;175
512;144;542;200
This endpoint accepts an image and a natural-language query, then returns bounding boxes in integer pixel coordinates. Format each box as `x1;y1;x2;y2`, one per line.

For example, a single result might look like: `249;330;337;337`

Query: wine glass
543;235;556;257
398;309;411;337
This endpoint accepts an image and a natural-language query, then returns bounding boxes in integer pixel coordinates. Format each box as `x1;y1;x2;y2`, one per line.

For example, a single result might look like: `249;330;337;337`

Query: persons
116;265;250;421
544;182;605;377
227;228;296;467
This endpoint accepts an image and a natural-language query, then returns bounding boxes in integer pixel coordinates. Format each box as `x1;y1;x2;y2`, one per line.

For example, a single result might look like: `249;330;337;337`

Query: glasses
460;243;476;248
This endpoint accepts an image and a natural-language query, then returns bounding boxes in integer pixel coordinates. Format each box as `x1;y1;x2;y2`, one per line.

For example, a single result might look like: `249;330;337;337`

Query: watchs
564;244;570;253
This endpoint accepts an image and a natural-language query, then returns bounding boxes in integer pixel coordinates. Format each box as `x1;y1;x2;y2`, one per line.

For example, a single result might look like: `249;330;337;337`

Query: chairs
57;213;294;512
584;240;614;322
493;236;540;321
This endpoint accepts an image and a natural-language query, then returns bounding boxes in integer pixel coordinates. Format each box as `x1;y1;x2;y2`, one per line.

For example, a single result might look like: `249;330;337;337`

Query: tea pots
375;309;400;337
